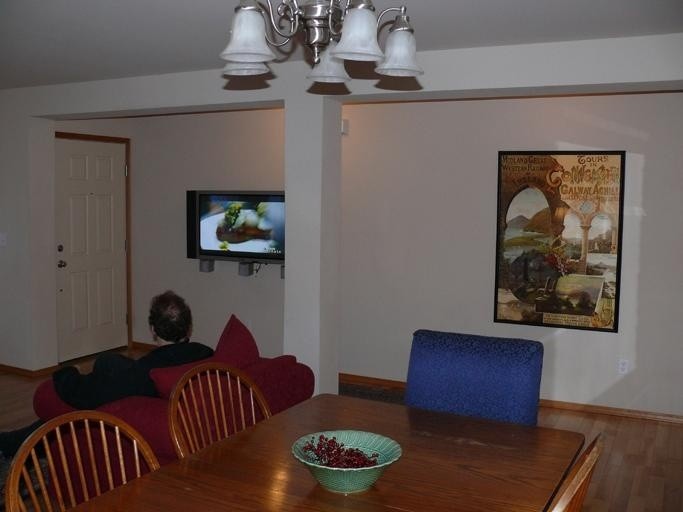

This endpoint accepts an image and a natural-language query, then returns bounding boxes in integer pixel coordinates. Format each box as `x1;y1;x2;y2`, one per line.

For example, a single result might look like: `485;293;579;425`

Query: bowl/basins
284;428;403;496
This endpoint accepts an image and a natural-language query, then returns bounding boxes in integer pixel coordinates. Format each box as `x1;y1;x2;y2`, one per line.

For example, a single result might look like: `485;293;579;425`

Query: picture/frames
493;151;626;332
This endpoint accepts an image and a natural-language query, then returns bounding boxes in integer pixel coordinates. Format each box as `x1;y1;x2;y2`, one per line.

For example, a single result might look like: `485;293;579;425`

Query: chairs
5;408;158;512
168;363;275;459
405;330;544;433
32;314;315;492
547;432;608;511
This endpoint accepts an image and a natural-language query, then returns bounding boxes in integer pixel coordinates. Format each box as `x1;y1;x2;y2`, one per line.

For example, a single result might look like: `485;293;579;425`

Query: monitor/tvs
195;191;285;266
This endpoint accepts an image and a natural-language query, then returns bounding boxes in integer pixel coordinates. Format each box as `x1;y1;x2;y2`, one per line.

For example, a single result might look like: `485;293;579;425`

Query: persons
1;290;216;458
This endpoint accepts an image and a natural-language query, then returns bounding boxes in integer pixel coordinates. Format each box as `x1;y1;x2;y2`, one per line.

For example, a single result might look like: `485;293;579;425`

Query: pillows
150;316;263;393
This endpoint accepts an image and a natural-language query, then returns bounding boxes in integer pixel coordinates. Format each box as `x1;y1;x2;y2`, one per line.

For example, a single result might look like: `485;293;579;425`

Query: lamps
219;1;426;83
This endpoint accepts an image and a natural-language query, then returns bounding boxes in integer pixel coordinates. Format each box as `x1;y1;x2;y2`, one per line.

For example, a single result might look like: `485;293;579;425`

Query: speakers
186;189;197;258
